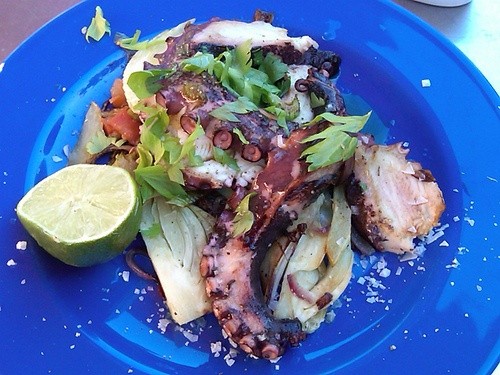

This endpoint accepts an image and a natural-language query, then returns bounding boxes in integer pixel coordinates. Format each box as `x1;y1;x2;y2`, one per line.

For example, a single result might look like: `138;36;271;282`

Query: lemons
15;163;144;268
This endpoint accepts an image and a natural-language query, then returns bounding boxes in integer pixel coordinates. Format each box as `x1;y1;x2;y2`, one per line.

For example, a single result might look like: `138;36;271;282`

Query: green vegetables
85;5;373;240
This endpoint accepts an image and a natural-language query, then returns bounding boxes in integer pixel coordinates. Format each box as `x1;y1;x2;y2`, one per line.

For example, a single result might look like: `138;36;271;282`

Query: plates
0;0;500;375
